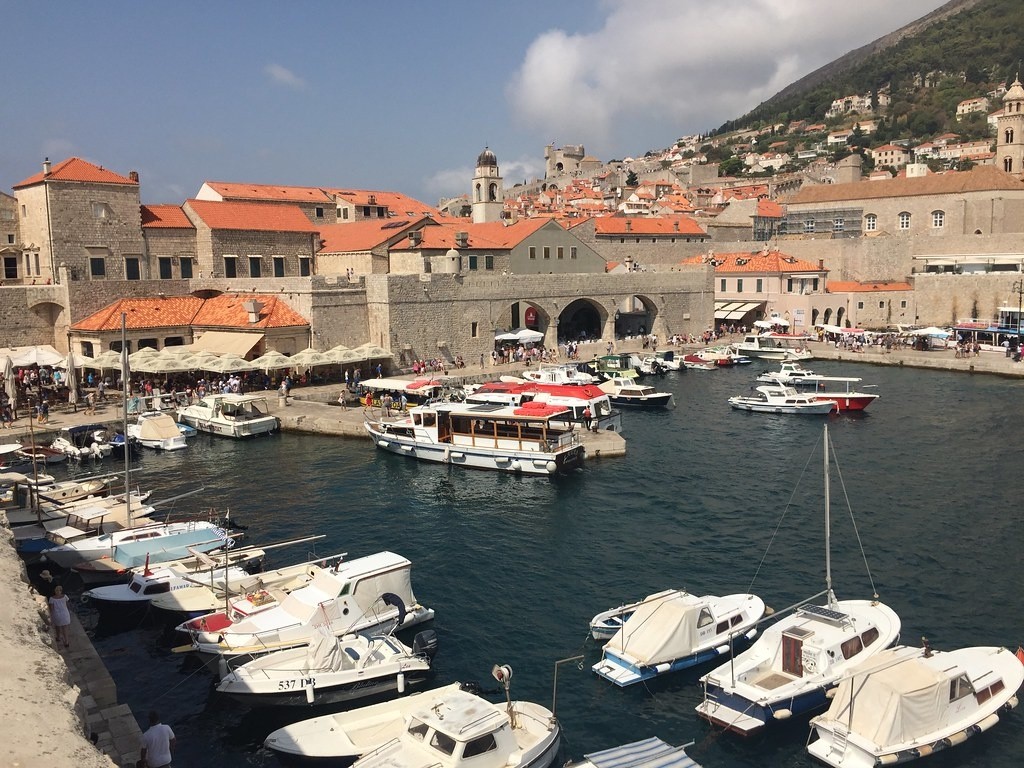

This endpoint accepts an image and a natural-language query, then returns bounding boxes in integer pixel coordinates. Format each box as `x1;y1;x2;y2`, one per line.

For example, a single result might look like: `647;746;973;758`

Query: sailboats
694;421;902;741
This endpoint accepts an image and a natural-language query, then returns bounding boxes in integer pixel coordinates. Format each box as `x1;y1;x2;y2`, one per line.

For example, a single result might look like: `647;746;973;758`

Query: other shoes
89;412;91;415
84;412;86;415
93;413;95;415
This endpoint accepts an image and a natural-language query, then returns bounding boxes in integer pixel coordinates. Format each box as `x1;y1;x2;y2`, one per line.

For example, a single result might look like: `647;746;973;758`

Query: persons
669;323;746;347
605;342;614;355
758;323;790;335
584;406;591;430
141;712;176;768
480;345;559;369
90;732;106;754
558;329;596;357
803;328;980;359
38;570;70;646
0;363;382;424
641;334;657;351
1001;339;1024;363
364;388;424;411
414;355;464;376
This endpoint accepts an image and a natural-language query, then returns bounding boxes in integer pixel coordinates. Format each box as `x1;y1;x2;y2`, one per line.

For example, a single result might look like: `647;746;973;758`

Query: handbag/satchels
338;398;343;404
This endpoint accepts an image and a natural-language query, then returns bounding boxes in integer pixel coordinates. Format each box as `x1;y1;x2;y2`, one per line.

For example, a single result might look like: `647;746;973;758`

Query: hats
236;376;239;378
230;375;233;377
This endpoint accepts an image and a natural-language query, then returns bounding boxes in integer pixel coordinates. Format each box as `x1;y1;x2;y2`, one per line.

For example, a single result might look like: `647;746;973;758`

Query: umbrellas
65;353;78;411
913;327;953;345
753;317;790;327
120;348;132;398
0;343;394;393
495;328;545;344
4;355;17;419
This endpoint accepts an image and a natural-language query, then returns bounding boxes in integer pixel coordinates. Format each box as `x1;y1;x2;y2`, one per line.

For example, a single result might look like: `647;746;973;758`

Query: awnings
714;302;762;320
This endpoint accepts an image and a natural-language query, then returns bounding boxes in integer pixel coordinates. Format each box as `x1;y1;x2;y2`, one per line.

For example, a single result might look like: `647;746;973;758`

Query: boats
626;348;752;375
69;519;245;591
0;444;153;534
728;378;837;415
588;601;642;640
791;377;880;410
749;360;861;389
354;665;562;768
148;544;436;681
586;355;640;380
263;675;483;768
592;587;767;688
522;362;594;387
16;408;198;464
814;320;960;348
363;397;585;476
728;332;815;360
804;640;1024;768
466;382;624;434
815;280;1024;352
356;378;482;411
175;393;278;440
10;313;204;557
597;376;672;405
215;593;439;720
499;376;530;383
